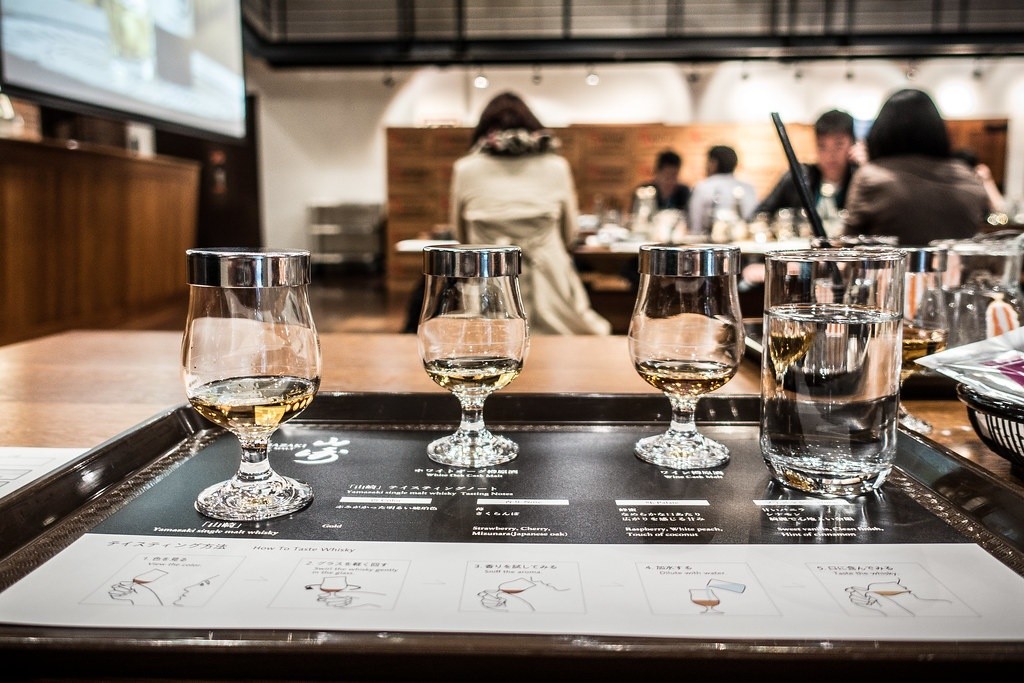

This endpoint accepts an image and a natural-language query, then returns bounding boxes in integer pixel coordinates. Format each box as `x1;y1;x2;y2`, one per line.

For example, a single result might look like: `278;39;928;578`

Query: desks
395;232;640;270
0;329;1024;493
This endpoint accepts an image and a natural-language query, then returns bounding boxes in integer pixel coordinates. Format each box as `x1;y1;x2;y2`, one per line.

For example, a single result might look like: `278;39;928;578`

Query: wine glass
627;242;746;470
418;243;529;468
181;247;322;519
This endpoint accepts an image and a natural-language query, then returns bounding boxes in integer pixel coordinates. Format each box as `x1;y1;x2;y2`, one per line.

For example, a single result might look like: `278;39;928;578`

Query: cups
943;242;1023;296
899;246;949;366
759;249;906;498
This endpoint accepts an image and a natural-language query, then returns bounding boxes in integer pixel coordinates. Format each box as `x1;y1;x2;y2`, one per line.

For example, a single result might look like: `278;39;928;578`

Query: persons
626;150;693;313
951;144;1020;219
450;92;612;336
834;88;1006;245
750;109;869;219
689;145;760;235
